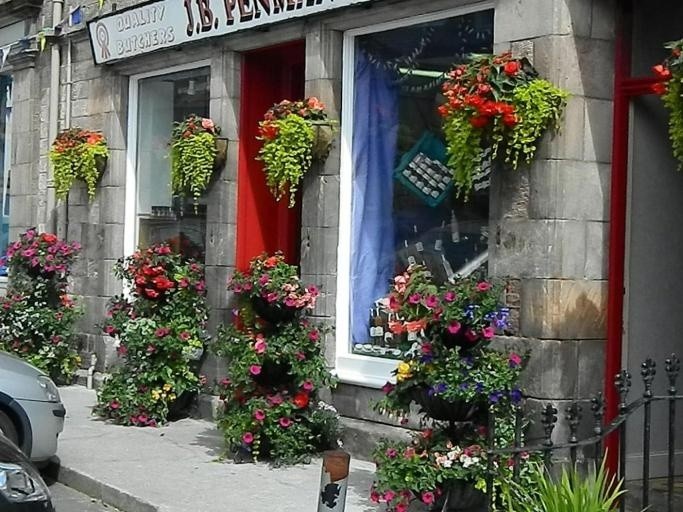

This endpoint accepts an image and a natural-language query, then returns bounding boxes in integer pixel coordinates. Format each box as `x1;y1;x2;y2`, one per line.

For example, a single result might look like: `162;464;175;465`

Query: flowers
0;224;83;386
369;261;546;512
204;250;338;465
86;241;208;428
162;112;222;218
253;96;325;209
46;127;108;205
650;38;683;175
436;49;571;205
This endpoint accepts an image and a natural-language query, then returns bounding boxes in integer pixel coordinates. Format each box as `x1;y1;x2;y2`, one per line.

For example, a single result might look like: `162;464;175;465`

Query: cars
0;350;66;512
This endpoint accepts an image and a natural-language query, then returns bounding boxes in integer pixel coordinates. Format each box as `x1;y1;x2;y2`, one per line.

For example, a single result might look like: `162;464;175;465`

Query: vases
71;155;106;183
212;135;225;170
309;119;332;156
